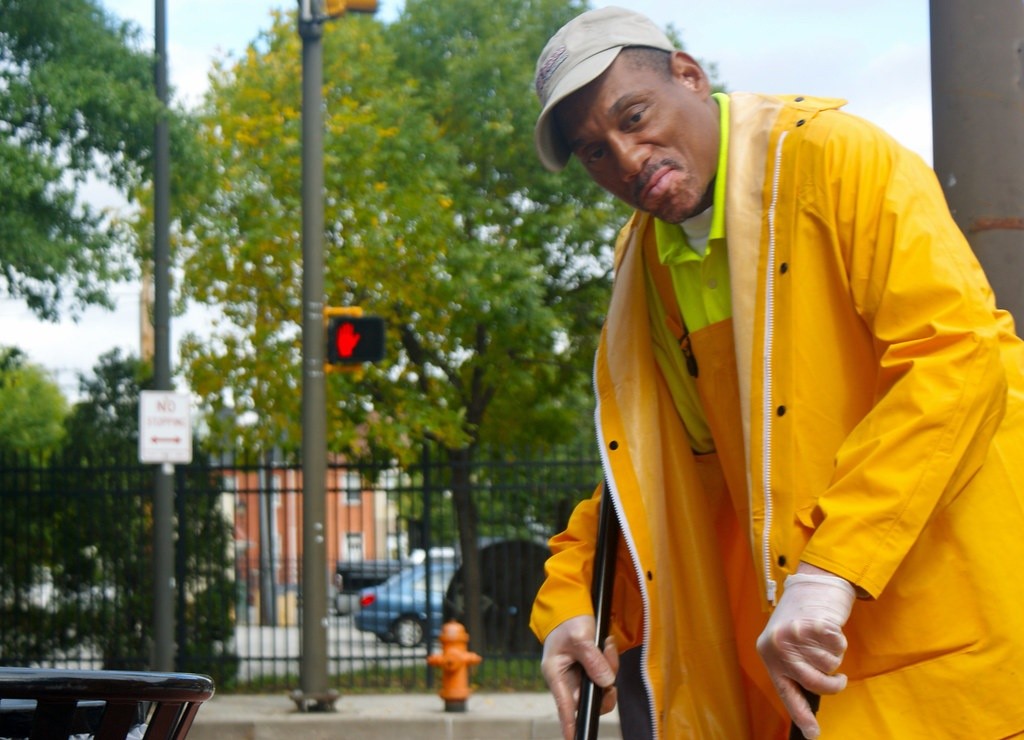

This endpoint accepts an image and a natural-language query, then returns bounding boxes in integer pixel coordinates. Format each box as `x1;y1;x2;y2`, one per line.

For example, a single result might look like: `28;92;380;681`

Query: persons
528;7;1024;740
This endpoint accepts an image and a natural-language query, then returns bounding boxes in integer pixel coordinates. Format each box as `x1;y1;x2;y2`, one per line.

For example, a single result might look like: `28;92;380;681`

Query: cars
332;552;461;650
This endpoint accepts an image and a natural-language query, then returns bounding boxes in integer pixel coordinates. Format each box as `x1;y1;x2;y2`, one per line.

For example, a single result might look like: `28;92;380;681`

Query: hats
533;7;677;174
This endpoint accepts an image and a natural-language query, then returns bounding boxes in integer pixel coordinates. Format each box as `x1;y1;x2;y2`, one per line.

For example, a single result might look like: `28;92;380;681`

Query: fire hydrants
428;618;482;717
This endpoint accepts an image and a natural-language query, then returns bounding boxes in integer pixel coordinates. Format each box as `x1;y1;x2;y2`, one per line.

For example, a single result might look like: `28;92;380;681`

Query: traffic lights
328;313;387;364
